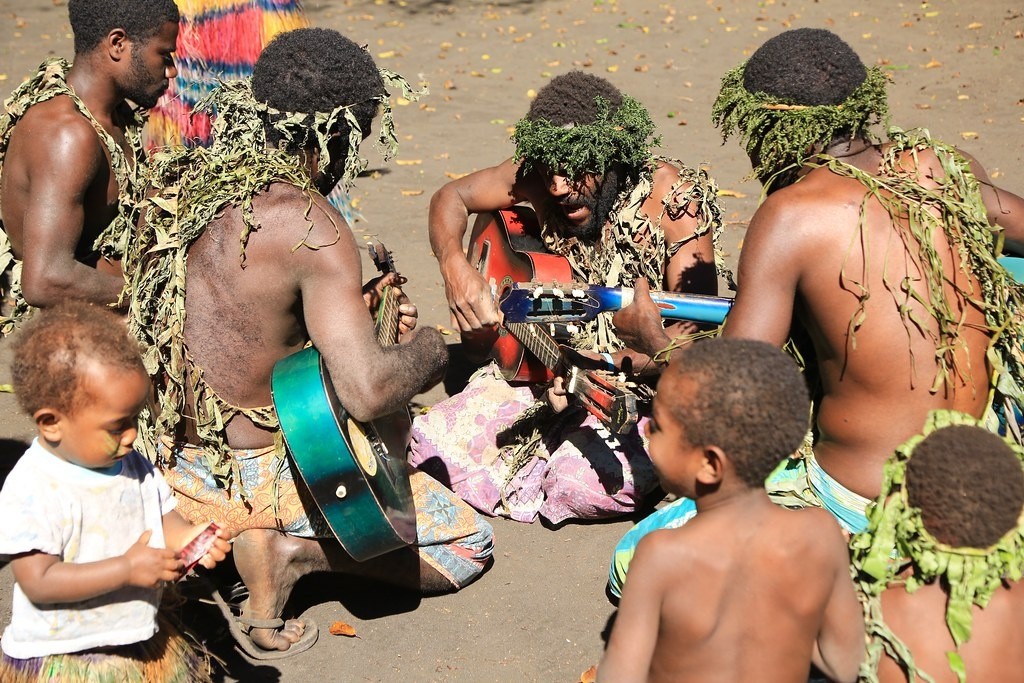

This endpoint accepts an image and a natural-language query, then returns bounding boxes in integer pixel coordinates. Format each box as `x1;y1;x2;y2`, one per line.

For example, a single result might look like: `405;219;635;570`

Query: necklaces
834;138;872;158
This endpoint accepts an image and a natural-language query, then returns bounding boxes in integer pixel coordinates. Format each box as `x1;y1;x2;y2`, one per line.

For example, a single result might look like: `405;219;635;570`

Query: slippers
192;564;318;660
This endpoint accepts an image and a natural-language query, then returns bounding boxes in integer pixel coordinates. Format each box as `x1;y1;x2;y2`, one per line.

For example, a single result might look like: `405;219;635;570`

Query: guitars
496;270;739;325
459;205;641;436
267;240;418;563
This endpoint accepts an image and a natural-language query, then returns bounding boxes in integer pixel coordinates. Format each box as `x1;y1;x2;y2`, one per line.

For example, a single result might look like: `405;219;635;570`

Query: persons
410;70;719;524
132;1;496;661
865;424;1022;683
596;337;864;683
609;27;1024;600
0;301;230;683
0;0;178;341
